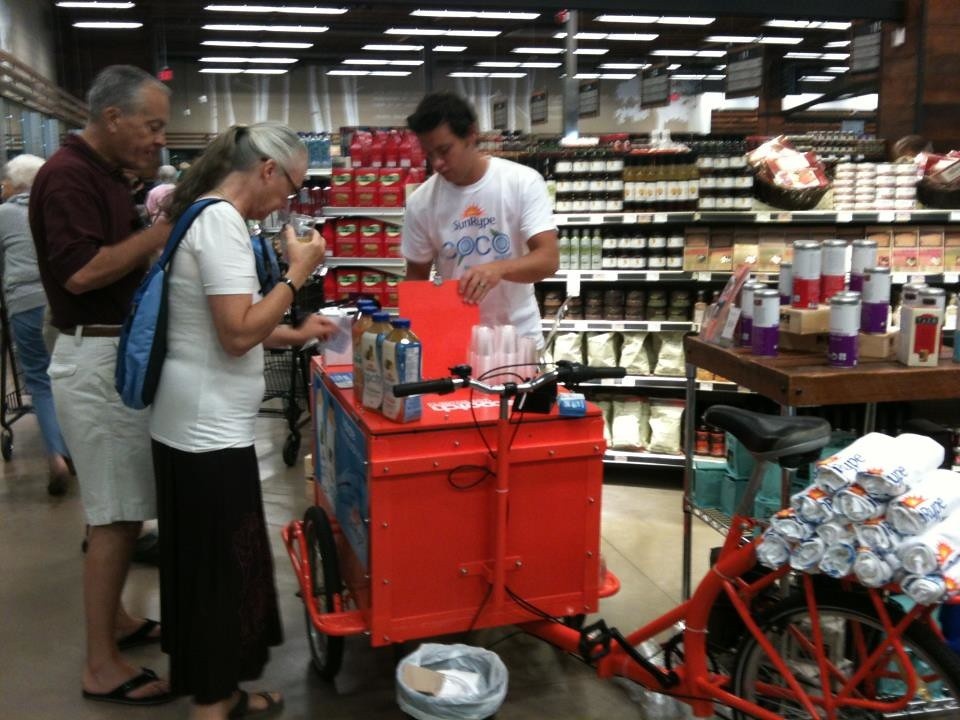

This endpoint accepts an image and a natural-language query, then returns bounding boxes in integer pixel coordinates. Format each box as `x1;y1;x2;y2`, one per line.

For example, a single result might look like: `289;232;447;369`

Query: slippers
116;617;162;652
83;666;177;705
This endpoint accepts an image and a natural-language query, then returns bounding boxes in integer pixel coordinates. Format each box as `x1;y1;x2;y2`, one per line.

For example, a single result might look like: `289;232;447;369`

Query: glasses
261;157;300;200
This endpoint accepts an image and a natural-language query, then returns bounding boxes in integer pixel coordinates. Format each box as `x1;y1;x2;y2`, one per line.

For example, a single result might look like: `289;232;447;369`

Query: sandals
228;690;285;720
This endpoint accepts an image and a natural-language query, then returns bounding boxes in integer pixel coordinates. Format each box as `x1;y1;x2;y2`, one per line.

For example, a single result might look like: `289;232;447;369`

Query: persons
403;92;560;369
149;122;339;720
124;162;189;226
0;154;75;496
27;64;174;705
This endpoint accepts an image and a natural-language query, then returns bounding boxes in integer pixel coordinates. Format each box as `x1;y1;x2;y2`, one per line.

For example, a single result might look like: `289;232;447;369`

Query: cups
282;213;316;265
739;238;946;364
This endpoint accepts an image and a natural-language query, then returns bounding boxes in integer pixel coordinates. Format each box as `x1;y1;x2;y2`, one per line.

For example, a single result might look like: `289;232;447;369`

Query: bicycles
388;358;960;719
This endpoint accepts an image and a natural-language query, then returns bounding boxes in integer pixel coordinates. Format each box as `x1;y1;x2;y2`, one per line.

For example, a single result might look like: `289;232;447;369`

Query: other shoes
48;476;68;497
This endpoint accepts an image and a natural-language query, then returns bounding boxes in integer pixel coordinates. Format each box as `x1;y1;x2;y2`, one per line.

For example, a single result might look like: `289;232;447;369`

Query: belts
60;325;124;338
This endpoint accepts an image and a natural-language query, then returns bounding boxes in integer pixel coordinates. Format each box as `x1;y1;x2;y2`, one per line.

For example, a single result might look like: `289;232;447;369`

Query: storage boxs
692;432;842;537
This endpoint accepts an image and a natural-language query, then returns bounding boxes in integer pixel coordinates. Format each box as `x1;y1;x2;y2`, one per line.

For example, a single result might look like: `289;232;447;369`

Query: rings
479;282;486;288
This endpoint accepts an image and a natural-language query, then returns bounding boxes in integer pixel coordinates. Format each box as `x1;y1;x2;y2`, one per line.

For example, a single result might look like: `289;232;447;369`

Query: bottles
784;129;866;160
350;300;394;411
557;229;683;270
382;318;424;423
476;130;753;214
698;421;726;459
296;130;333;168
545;290;719;323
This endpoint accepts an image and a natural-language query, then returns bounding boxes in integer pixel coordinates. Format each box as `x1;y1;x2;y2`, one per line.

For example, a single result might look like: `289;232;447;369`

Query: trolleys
0;302;37;461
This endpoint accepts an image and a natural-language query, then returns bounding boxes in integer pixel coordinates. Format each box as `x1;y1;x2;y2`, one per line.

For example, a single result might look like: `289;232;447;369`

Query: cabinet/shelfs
318;205;960;489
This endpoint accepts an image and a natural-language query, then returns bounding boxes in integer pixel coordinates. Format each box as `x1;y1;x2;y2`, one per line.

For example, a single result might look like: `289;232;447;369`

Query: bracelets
280;278;298;298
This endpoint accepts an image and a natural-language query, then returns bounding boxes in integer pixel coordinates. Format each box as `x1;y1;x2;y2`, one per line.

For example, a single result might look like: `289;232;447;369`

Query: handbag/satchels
114;263;168;409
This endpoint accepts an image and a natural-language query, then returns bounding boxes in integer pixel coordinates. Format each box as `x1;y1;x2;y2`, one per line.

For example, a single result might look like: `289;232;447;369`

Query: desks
682;335;960;598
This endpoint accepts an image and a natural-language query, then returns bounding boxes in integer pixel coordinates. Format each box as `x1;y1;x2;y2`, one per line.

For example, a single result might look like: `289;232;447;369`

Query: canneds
751;289;781;356
833;161;920;211
861;266;891;333
790;240;822;311
849;240;879;292
778;261;792;305
820;239;848;304
827;291;862;368
697;153;754;211
542;289;690;322
602;229;685;270
902;283;946;357
554;146;624;214
740;283;768;349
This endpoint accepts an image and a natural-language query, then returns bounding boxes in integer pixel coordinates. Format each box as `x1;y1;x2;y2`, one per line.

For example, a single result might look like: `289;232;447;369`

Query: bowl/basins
832;161;921;210
